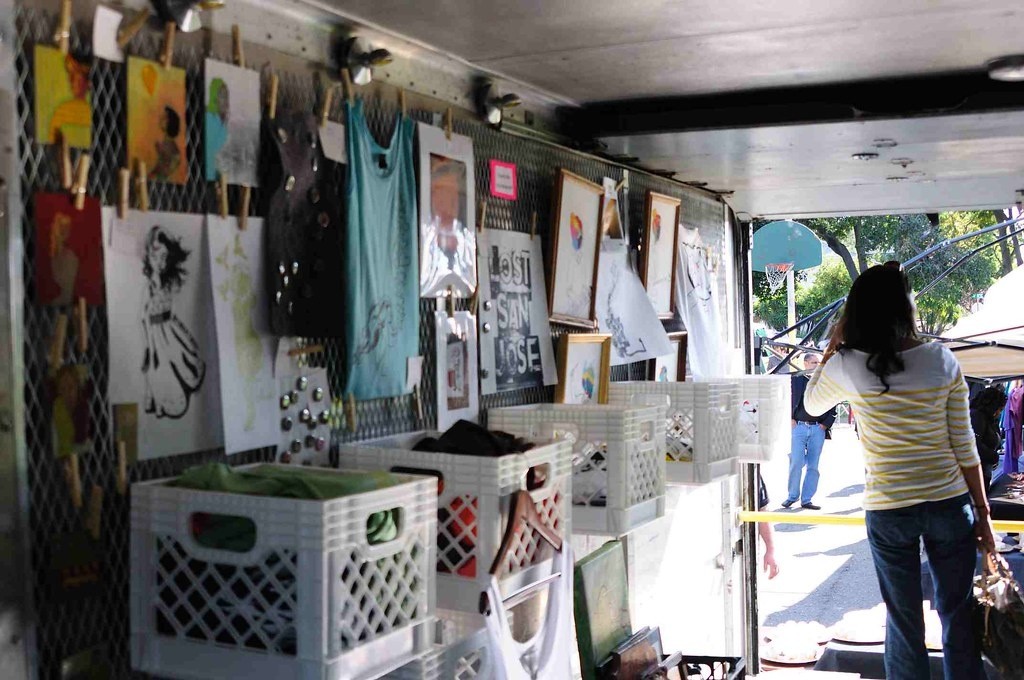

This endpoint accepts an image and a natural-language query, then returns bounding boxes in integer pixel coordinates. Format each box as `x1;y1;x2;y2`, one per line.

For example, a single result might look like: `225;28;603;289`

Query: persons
782;354;837;510
969;384;1008;500
758;474;780;579
804;261;996;680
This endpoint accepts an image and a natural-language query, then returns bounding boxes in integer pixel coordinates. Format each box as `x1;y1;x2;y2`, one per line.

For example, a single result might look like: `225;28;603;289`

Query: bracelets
971;503;989;509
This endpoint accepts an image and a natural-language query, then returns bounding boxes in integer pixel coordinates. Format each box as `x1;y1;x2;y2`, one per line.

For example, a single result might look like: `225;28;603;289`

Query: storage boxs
131;382;736;680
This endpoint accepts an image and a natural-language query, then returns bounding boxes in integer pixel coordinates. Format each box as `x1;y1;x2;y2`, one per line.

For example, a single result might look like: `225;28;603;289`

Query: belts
799;421;819;426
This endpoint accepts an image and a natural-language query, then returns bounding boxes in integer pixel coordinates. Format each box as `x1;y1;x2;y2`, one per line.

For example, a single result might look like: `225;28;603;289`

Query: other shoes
782;498;799;508
802;503;821;510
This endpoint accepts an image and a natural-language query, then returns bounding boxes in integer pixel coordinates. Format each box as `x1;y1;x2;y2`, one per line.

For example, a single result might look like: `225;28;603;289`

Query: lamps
476;82;517;125
154;0;224;31
340;34;391;87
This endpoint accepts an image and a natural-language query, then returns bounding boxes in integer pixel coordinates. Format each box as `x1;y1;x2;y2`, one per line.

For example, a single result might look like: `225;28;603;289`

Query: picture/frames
639;189;681;320
548;166;606;329
648;331;688;381
554;333;613;405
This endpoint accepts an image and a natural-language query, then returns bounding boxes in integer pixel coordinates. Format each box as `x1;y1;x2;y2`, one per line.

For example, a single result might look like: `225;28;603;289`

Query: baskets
608;375;791;484
338;430;572;615
488;402;666;535
129;463;439;680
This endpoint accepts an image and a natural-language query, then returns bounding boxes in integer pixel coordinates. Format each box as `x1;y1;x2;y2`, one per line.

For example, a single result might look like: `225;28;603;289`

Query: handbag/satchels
973;542;1024;673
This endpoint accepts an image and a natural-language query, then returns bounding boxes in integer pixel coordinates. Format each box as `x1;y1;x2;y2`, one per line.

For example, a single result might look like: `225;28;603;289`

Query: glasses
884;261;900;276
